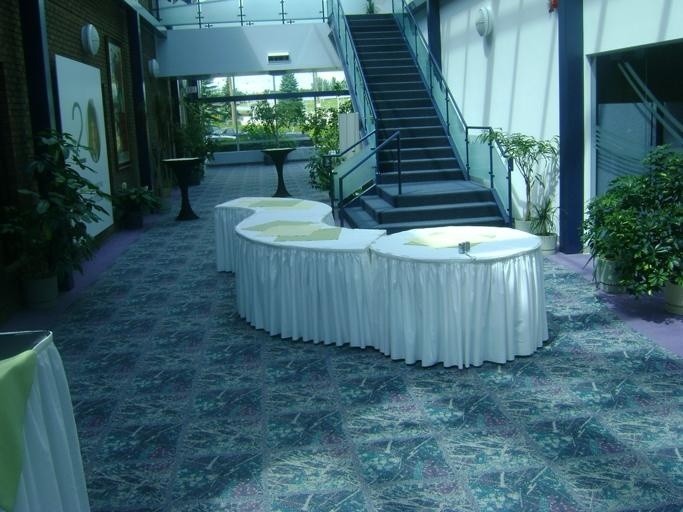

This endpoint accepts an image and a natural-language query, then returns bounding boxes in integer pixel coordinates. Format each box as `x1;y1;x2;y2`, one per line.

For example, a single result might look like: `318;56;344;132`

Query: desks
368;223;552;371
160;155;205;223
214;193;387;349
258;147;298;198
0;328;90;512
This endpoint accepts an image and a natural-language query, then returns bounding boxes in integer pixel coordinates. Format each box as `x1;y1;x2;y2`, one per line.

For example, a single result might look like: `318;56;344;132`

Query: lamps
266;52;290;65
79;22;101;57
146;58;160;80
474;6;493;40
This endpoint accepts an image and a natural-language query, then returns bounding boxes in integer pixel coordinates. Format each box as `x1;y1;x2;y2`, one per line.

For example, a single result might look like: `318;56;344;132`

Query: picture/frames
102;32;133;171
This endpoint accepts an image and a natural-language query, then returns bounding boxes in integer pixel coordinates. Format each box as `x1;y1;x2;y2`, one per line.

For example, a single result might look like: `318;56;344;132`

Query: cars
202;123;310;145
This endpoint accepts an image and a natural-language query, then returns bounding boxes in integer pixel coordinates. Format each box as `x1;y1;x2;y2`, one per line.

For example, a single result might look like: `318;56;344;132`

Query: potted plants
528;195;567;255
462;127;563;231
236;72;311;166
114;182;169;231
0;123;113;308
574;145;683;300
158;82;236;187
659;152;683;318
